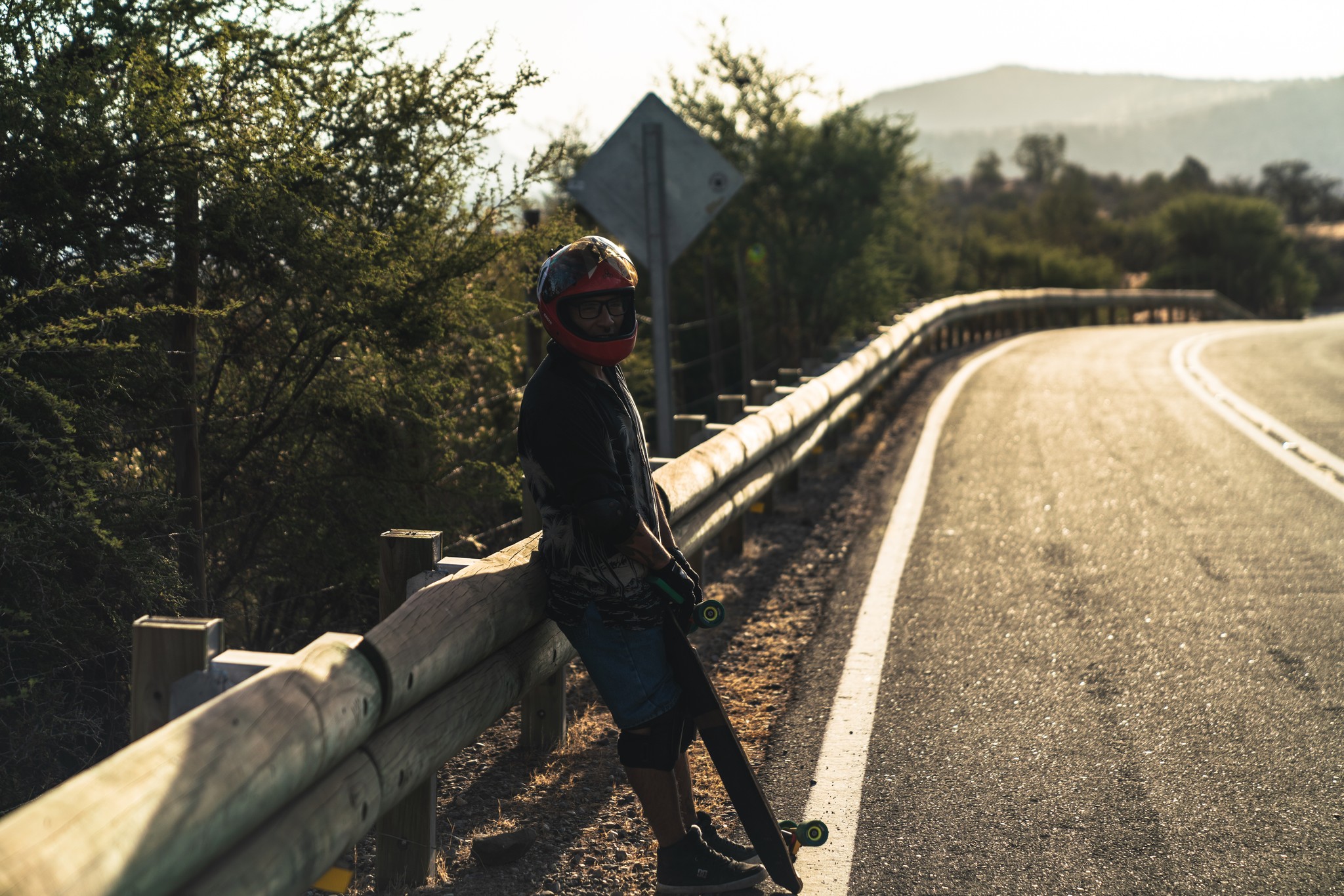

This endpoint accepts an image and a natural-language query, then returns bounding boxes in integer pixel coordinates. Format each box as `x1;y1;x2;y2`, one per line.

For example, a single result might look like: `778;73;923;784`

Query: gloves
651;558;696;636
667;547;702;604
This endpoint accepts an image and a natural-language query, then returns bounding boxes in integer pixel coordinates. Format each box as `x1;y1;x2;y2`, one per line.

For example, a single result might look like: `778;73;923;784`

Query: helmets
536;233;639;364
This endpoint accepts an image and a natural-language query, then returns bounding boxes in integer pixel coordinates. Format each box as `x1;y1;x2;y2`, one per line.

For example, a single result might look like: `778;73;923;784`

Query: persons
515;235;769;894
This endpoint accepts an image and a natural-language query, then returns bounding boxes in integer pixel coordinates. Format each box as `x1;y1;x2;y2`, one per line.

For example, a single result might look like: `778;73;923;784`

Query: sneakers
687;809;764;864
653;825;768;893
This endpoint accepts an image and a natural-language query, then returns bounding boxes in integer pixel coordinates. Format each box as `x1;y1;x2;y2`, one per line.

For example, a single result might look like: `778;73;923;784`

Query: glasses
568;296;630;319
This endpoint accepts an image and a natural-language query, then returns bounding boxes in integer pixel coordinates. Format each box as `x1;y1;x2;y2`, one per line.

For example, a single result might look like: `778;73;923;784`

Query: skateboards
663;599;828;894
